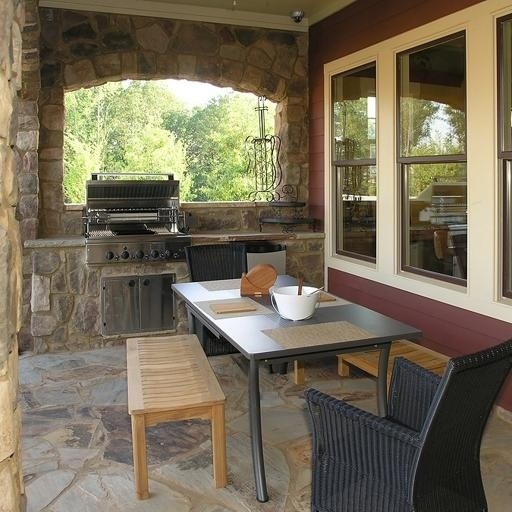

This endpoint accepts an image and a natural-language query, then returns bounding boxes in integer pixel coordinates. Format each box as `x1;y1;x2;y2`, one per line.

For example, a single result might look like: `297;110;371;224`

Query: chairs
245;241;287;276
305;339;511;506
185;243;244;282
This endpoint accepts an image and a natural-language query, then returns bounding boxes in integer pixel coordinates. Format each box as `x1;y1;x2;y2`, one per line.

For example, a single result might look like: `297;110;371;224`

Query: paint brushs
296;270;305;294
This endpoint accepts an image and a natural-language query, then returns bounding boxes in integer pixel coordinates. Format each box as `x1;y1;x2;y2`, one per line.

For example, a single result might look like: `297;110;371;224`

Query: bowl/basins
271;286;320;321
447;225;467;248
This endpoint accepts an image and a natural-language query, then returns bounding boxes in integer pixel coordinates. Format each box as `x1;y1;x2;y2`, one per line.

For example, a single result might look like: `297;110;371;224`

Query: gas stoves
419;206;467;225
85;213;189;264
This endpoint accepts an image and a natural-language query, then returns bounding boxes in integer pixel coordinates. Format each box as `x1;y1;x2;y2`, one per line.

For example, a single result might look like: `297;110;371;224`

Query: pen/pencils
307;286;324;296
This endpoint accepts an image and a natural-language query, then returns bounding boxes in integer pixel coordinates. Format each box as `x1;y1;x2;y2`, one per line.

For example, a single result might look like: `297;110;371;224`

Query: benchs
338;340;453;384
124;335;226;498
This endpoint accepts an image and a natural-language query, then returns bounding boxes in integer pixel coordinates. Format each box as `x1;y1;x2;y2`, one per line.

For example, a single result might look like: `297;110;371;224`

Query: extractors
417;184;467;205
85;173;181;213
